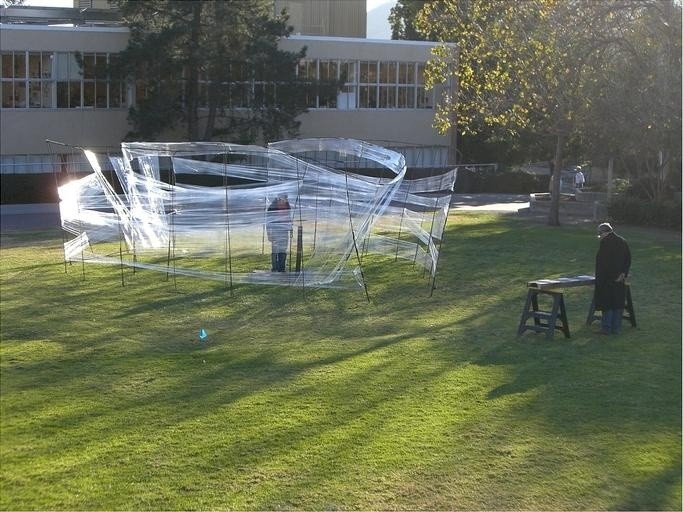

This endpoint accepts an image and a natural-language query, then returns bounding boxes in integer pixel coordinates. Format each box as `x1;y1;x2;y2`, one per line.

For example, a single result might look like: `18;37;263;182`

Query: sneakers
592;326;621;335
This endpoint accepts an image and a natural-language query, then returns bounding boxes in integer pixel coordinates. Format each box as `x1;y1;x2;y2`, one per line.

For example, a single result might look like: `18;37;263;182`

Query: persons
593;222;632;338
265;192;293;273
574;166;586;191
